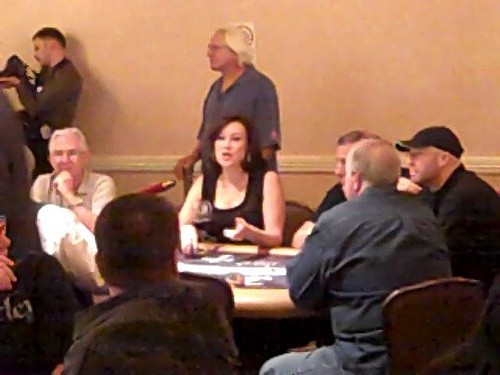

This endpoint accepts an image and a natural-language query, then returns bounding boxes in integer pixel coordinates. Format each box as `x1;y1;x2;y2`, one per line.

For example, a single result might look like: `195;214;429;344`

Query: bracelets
68;201;84;207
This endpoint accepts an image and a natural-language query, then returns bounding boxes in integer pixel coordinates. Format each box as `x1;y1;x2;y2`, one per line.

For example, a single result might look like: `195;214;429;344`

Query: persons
0;26;83;183
0;85;500;375
173;26;283;180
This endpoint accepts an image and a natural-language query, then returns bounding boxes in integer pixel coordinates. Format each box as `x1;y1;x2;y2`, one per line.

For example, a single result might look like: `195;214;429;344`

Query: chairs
379;275;489;375
283;199;315;247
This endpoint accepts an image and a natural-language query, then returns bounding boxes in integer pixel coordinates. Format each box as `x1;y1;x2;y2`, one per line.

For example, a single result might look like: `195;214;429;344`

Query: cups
180;225;198;253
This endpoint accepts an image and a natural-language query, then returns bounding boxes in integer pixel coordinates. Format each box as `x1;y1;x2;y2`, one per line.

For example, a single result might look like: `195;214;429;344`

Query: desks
173;239;313;320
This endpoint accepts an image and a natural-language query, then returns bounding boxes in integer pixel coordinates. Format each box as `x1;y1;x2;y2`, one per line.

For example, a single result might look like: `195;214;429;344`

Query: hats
396;127;463;159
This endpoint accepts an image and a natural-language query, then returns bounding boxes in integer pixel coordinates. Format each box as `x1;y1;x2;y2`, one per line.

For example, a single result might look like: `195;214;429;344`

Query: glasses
208;44;230;52
